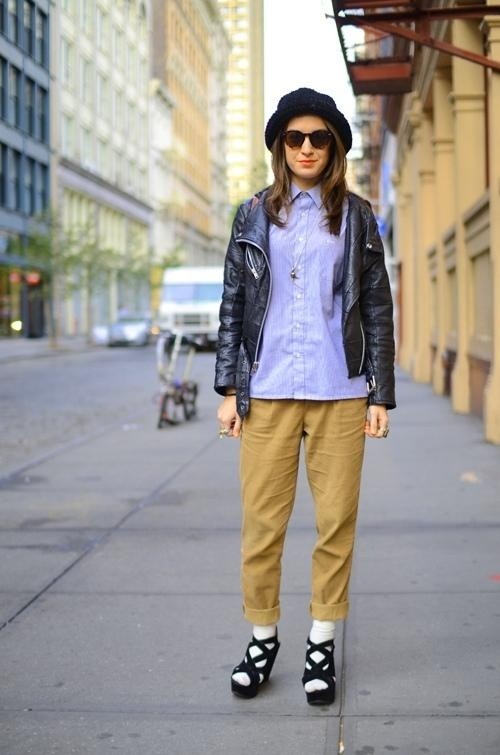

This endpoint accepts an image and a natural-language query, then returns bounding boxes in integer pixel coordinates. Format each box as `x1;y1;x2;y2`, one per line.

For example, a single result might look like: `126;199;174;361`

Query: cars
93;311;151;346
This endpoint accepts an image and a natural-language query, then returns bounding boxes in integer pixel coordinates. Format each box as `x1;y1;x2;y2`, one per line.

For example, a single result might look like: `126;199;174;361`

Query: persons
213;88;397;706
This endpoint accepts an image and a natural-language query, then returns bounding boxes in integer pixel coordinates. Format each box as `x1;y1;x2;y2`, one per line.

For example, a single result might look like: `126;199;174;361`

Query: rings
219;429;230;439
378;427;389;438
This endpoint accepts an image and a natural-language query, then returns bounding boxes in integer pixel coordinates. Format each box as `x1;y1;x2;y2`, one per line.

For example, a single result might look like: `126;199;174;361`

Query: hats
265;87;352;154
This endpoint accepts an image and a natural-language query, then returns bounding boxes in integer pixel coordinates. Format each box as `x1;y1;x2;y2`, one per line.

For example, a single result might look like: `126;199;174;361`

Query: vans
159;266;224;350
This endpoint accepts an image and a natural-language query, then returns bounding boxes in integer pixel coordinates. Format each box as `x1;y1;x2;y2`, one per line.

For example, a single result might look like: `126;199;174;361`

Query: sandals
230;626;280;698
302;637;336;705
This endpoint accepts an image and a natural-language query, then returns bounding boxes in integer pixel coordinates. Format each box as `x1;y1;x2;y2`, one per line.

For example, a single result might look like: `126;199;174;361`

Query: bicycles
153;322;205;429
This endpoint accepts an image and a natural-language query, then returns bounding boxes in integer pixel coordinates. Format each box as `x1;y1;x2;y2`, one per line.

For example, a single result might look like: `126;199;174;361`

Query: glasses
280;130;333;150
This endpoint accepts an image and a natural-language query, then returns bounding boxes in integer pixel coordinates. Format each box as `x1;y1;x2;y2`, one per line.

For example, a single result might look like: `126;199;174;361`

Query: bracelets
225;393;235;396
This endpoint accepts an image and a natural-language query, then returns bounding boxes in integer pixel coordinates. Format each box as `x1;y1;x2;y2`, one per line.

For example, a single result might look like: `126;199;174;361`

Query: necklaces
286;207;322;279
